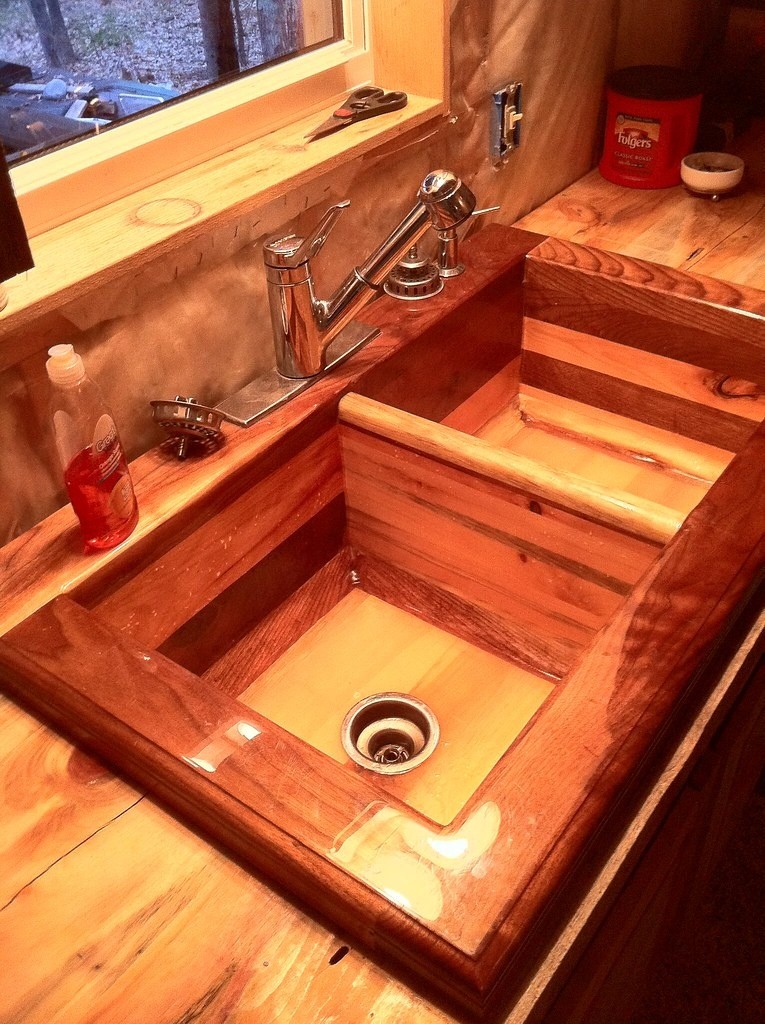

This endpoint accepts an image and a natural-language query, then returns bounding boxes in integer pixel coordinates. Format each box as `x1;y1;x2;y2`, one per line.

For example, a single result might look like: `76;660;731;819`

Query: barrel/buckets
597;64;703;190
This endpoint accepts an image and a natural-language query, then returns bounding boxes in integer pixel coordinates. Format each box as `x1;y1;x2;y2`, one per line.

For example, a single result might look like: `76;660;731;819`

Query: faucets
262;168;478;380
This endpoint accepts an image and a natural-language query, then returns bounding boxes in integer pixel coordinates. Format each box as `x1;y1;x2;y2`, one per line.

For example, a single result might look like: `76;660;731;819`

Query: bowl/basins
680;152;745;194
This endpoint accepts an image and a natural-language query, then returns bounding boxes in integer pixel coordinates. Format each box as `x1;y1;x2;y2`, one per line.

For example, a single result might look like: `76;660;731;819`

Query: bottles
46;343;138;552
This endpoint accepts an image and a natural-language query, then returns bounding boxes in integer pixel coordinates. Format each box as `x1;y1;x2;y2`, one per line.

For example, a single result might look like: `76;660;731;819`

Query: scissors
303;85;408;138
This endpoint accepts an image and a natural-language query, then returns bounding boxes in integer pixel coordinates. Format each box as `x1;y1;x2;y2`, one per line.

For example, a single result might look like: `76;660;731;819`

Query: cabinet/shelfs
537;662;764;1024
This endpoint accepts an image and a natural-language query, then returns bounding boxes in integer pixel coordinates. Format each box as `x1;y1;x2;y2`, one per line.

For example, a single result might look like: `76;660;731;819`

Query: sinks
61;389;686;831
349;252;765;517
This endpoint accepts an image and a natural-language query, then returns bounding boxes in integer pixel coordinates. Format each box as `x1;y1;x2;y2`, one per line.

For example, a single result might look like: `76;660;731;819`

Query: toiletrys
44;341;140;550
